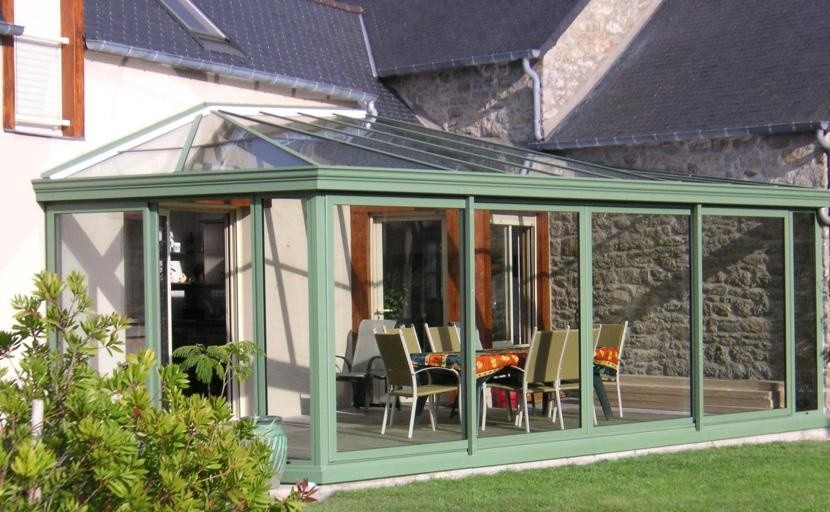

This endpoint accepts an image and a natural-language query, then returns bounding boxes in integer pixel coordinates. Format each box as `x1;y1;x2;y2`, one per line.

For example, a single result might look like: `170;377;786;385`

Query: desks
409;346;621;420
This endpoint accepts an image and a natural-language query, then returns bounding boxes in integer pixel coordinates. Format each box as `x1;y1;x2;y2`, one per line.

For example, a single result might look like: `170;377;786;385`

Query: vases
240;413;287;489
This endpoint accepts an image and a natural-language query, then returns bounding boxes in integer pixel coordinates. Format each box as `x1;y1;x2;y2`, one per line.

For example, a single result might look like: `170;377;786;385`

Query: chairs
337;318;399;410
382;321;423;354
548;325;602;428
482;323;568;432
372;327;462;438
592;320;631;418
425;320;463;353
